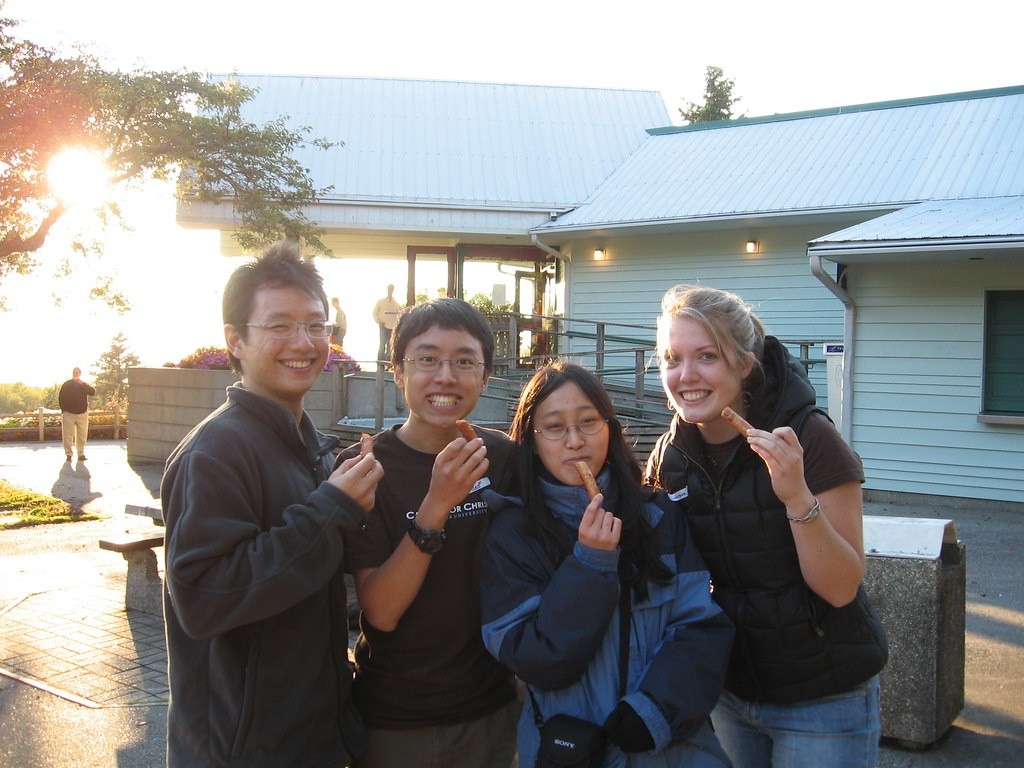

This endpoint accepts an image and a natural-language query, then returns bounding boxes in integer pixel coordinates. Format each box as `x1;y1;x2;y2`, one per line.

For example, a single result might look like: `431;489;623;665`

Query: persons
478;361;739;768
161;248;537;768
59;367;95;462
643;284;890;768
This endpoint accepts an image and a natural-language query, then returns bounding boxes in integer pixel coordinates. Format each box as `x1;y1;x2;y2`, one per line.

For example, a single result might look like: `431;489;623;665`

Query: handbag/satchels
535;713;606;768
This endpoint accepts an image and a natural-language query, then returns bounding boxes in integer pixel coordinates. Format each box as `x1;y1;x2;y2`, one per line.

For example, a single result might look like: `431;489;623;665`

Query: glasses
402;353;486;372
533;415;609;440
239;319;334;338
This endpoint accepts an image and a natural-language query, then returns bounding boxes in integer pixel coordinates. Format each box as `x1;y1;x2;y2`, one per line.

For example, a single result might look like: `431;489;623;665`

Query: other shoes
78;456;88;460
67;456;71;461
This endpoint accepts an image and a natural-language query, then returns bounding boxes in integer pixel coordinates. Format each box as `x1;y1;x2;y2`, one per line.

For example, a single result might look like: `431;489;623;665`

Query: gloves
602;698;653;753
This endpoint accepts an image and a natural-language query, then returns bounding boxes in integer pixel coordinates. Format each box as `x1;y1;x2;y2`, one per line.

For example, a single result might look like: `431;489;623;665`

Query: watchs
407;518;448;554
786;497;820;524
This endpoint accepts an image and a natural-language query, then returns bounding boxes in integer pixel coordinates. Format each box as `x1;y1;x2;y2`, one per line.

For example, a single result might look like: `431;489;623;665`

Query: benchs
100;528;166;619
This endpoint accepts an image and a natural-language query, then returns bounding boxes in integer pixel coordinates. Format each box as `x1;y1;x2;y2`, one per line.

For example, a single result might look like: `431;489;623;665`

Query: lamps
746;239;759;253
593;248;605;262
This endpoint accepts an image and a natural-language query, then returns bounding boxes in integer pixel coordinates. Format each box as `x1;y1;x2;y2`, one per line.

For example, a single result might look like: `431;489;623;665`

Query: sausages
360;433;374;458
455;419;478;441
573;461;600;501
721;407;755;437
